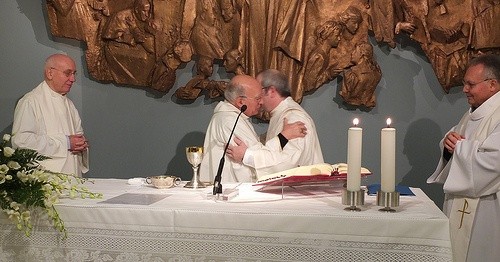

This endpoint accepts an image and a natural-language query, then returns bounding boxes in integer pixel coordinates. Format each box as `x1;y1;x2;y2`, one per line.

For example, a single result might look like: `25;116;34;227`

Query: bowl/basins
146;175;182;188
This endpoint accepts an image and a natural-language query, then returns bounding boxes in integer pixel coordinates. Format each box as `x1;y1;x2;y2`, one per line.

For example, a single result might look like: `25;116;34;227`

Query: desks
0;179;454;262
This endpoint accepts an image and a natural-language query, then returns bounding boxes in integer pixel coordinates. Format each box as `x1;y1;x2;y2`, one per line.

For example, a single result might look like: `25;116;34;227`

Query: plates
127;177;146;185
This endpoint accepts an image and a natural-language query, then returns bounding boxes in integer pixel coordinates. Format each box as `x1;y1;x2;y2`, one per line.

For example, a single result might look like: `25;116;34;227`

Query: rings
301;129;303;133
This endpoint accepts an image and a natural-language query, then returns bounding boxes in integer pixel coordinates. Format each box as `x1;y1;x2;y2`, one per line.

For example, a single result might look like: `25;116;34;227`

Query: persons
426;53;500;262
224;69;324;181
176;57;214;100
198;74;307;185
191;0;226;59
365;0;500;95
101;0;164;88
220;0;382;107
11;54;89;176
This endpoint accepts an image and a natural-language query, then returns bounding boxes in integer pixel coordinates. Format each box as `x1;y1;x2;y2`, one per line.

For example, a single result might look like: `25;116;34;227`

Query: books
254;163;371;184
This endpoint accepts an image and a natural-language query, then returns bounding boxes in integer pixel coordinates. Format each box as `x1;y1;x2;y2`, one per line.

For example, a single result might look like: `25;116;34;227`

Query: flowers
0;133;103;240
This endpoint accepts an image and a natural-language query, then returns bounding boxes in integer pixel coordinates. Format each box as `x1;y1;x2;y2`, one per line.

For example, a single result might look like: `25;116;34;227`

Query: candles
380;118;395;192
347;118;363;191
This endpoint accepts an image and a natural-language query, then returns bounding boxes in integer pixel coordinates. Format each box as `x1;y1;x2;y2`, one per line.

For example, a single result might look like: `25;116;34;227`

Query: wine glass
186;146;206;188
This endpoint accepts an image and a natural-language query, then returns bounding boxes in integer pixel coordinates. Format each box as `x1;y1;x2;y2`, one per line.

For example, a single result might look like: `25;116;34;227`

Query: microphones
213;104;247;194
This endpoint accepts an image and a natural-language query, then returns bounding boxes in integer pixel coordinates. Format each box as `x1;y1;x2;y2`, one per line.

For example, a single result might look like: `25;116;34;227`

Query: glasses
240;96;261;104
50;67;77;77
462;78;493;90
